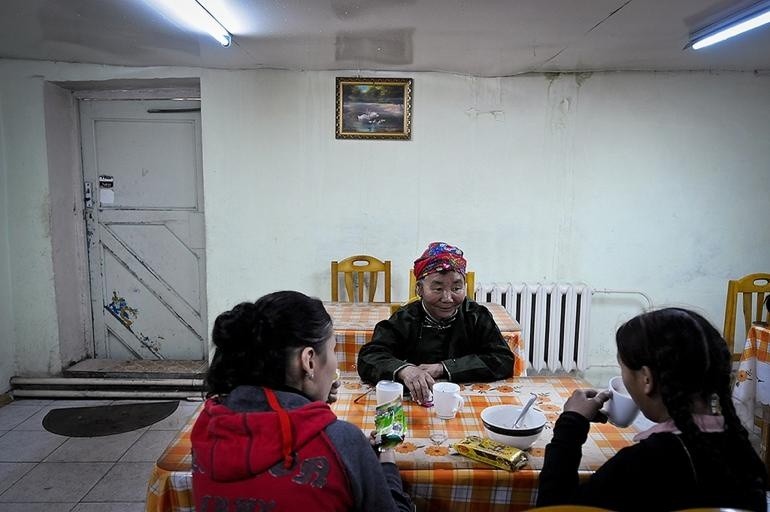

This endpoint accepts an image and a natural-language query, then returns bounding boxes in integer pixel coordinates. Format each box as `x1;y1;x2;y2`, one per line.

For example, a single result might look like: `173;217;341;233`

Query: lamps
146;0;232;49
683;0;770;53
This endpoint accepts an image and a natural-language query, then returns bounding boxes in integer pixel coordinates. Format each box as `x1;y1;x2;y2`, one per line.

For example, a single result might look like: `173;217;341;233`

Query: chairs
331;256;391;303
409;269;474;302
724;274;770;461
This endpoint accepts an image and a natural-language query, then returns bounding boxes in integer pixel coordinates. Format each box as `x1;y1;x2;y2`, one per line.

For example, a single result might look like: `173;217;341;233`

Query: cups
375;380;404;409
599;378;640;428
433;380;466;420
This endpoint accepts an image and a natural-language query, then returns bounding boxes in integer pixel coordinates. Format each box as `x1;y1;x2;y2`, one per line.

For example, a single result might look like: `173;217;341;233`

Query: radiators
478;282;591;373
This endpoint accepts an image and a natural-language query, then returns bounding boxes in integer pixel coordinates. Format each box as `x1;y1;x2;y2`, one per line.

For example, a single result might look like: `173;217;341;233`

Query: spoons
507;394;539;428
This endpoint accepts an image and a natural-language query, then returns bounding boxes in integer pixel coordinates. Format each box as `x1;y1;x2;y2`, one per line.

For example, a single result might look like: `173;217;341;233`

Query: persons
192;290;417;511
356;241;515;404
536;307;770;512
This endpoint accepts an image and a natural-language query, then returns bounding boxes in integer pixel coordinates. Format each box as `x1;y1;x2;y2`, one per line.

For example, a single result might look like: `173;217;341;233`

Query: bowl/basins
479;406;546;448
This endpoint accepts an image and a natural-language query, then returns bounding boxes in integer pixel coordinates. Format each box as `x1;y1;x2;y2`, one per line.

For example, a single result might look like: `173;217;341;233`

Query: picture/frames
335;76;413;142
